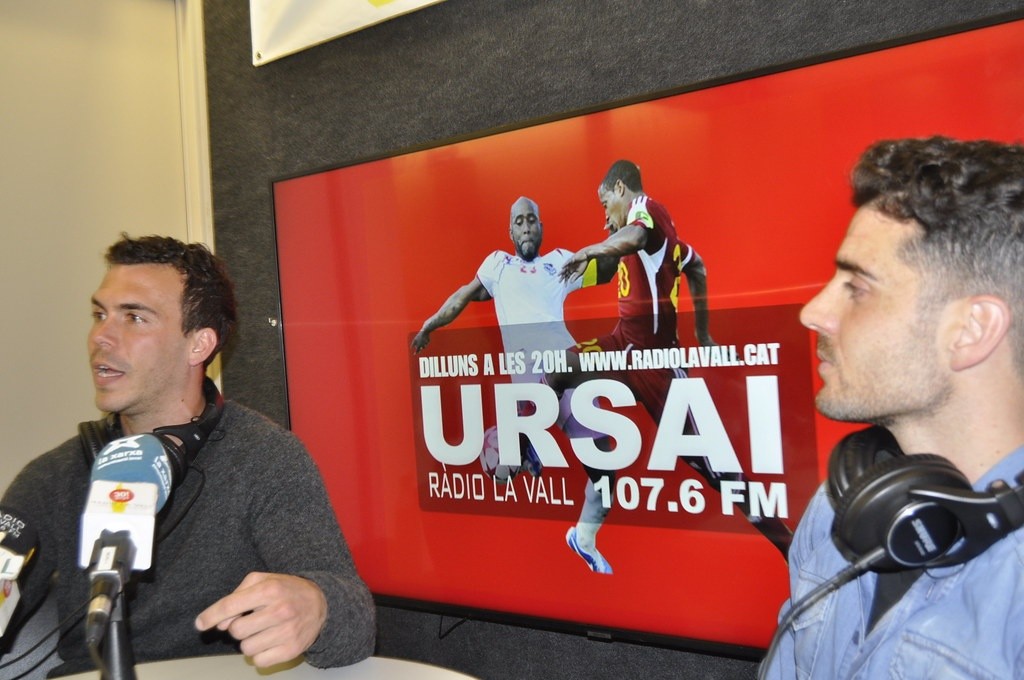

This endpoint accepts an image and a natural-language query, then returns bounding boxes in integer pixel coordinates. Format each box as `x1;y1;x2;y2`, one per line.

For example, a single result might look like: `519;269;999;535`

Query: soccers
479;425;521;483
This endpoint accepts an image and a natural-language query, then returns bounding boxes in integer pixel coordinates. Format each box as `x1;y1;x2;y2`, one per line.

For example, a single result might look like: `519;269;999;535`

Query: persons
0;236;377;680
495;160;794;579
412;195;622;573
752;135;1024;680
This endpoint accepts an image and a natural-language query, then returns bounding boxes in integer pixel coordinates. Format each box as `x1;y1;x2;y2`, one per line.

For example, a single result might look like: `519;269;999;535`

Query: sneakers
523;439;543;478
565;526;614;576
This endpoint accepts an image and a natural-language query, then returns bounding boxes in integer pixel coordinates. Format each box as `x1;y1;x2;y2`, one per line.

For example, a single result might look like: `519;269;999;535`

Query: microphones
0;505;39;635
79;433;173;647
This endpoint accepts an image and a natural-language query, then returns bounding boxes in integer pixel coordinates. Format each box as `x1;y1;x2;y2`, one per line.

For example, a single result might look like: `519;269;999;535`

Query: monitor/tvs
269;7;1024;661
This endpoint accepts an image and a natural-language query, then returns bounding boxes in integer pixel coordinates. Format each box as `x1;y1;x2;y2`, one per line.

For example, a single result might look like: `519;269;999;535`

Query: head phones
826;423;1024;574
77;375;224;487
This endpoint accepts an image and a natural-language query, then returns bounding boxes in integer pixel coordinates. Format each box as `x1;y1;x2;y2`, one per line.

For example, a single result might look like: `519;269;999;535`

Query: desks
57;654;476;680
